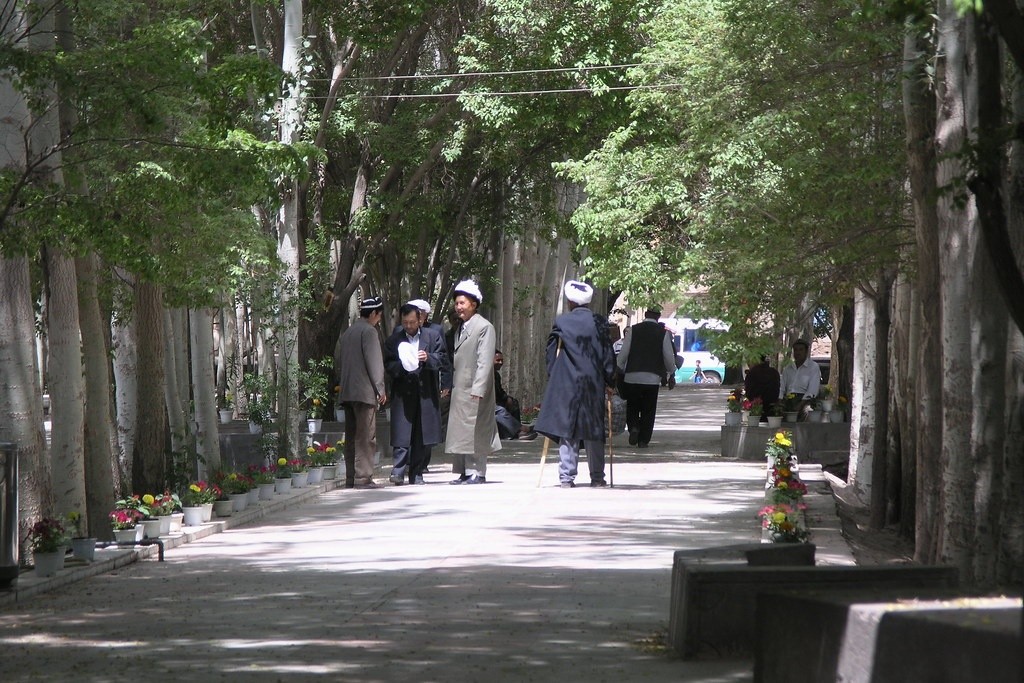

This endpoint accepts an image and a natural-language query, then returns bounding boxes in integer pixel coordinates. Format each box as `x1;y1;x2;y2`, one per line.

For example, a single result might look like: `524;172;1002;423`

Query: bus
659;318;751;384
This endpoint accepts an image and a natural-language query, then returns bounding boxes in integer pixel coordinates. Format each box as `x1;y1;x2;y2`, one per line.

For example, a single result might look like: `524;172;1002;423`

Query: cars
810;356;831;398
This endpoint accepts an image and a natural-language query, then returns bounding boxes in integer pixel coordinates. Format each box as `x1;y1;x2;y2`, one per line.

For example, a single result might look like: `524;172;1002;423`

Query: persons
689;360;707;383
533;280;616;489
334;297;387;489
405;299;453;473
658;322;677;386
383;304;447;486
444;280;502;485
494;349;538;440
616;308;675;447
613;326;631;355
445;305;462;352
778;339;820;411
745;355;781;413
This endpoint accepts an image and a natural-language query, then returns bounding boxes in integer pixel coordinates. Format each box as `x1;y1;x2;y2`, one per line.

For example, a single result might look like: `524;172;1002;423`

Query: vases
34;552;59;578
808;411;822;423
221;393;235;411
336;410;345;422
307;418;323;433
767;416;783;428
784;412;799;422
56;546;67;569
726;413;742;425
747;416;761;427
820;400;832;412
831;410;844;423
219;410;233;424
71;538;97;561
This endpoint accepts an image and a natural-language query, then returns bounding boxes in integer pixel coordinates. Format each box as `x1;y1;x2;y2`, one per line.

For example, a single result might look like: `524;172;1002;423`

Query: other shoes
345;481;378;489
409;481;425;485
389;474;404;485
560;481;576;488
519;432;538;440
590;479;607;488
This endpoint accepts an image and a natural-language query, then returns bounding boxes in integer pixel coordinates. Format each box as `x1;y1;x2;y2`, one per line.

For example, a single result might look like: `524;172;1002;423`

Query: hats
792;339;811;347
407;299;431;314
398;342;419;372
453;279;482;309
565;280;594;305
360;297;382;309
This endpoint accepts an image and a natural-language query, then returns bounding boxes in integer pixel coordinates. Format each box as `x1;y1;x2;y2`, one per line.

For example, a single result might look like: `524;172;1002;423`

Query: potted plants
766;401;785;428
249;403;272;434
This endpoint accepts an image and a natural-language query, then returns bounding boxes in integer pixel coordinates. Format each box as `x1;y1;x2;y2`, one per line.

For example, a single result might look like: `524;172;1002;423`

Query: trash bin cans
0;443;20;589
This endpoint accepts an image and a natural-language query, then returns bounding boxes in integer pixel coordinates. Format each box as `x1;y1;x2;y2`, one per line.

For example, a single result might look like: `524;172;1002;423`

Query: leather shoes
462;474;486;485
448;474;472;485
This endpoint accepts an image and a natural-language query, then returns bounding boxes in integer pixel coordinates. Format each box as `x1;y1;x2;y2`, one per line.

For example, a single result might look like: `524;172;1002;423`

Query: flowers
727;396;742;412
782;392;801;412
332;386;344;410
757;429;810;543
818;384;834;400
520;403;543;423
28;440;345;552
805;395;818;410
308;399;323;418
835;396;847;410
740;396;764;416
